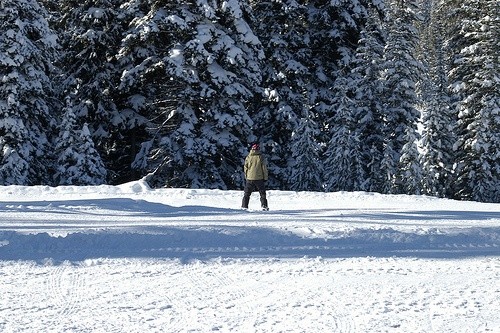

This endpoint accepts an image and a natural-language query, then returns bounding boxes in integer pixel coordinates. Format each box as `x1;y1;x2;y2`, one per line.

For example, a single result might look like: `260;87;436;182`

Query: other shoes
261;205;269;210
241;207;248;210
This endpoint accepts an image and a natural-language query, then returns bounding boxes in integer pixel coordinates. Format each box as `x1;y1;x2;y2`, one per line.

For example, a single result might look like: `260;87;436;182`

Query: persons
241;144;269;211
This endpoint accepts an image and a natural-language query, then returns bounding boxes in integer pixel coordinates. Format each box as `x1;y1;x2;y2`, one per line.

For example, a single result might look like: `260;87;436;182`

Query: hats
252;144;260;150
